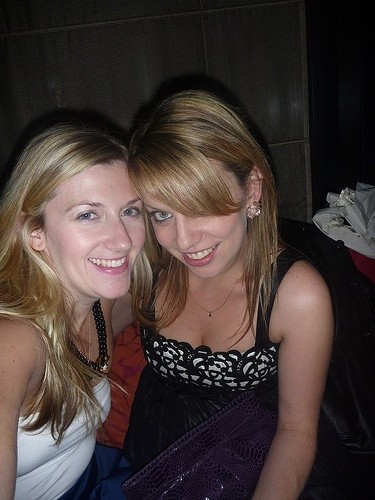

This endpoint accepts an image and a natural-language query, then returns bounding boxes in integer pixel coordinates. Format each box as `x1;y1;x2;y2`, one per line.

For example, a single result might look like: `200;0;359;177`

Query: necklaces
188;275;241;318
73;313;93;363
69;300;111;376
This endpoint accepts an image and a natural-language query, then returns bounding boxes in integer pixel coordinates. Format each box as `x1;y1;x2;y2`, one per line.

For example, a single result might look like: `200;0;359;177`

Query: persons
0;120;147;499
93;86;336;499
